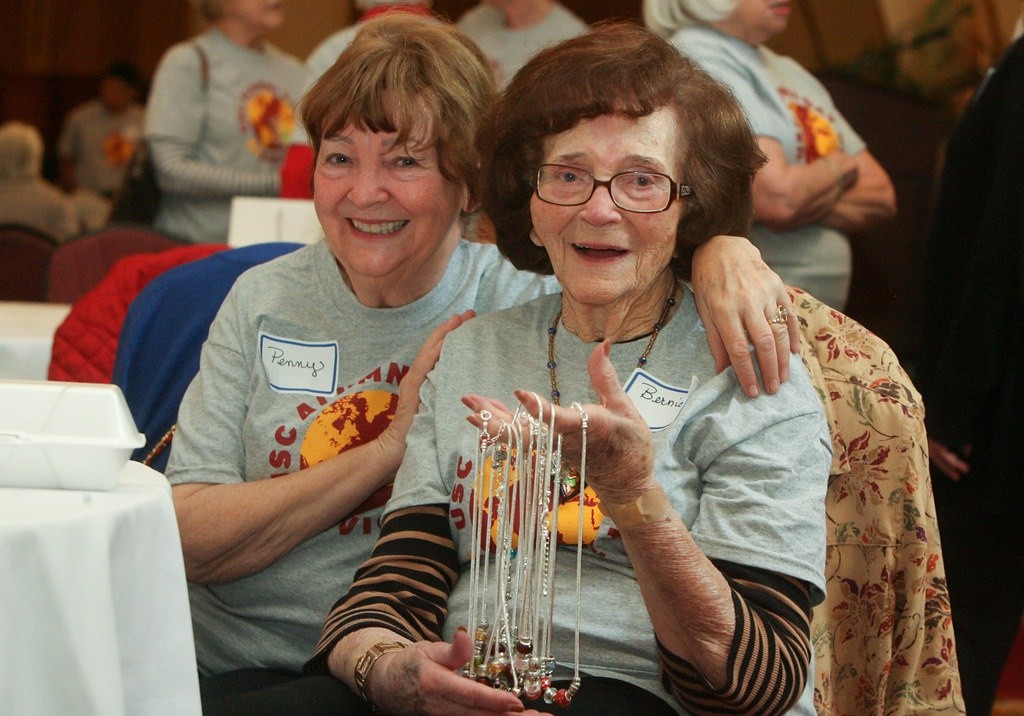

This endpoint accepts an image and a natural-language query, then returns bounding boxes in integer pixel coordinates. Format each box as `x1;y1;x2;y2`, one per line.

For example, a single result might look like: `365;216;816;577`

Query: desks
0;461;203;716
0;298;75;381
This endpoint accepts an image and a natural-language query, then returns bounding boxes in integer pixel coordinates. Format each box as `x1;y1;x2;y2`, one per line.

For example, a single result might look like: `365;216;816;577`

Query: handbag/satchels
108;142;165;230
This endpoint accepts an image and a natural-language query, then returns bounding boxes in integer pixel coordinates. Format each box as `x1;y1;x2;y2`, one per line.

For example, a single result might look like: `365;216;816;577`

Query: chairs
0;217;312;476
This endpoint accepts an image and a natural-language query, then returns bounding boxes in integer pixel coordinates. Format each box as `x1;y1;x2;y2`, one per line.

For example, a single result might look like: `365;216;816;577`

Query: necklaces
548;271;682;497
463;388;587;704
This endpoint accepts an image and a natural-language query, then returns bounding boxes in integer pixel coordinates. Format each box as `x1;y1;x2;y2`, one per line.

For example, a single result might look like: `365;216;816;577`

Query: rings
767;305;789;324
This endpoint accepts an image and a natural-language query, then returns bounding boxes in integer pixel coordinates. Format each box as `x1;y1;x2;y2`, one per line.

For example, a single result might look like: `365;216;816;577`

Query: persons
164;8;803;705
895;0;1022;713
307;0;433;82
450;0;592;91
638;0;895;313
144;0;317;245
0;58;149;243
200;18;832;712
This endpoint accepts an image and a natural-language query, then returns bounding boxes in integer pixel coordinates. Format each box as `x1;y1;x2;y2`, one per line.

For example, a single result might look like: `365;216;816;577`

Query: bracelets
352;641;418;704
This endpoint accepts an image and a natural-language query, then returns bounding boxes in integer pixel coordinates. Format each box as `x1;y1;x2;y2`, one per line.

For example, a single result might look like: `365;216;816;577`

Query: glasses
523;163;694;214
